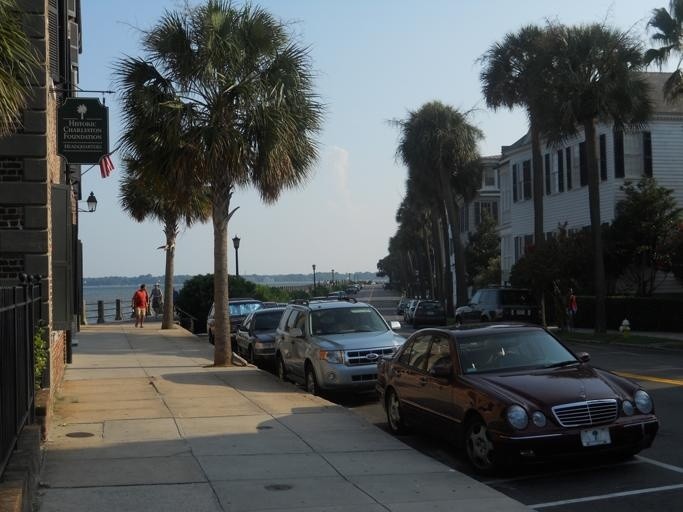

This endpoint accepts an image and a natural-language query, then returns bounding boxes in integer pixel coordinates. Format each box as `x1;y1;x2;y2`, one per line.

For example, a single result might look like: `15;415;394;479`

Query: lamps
75;189;98;213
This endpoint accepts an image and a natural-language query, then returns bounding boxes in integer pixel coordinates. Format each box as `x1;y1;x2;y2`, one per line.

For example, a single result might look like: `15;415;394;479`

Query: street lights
232;234;240;276
331;269;334;283
312;264;316;295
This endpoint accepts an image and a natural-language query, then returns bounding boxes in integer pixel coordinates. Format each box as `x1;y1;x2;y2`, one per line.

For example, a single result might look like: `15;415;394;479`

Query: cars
394;296;447;327
328;283;361;297
374;318;660;480
382;283;393;290
235;301;289;364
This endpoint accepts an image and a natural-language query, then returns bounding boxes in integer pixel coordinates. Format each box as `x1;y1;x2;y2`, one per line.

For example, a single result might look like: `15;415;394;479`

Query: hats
153;283;160;286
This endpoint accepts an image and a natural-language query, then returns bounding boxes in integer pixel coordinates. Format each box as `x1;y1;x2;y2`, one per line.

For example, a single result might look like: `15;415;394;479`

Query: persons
173;287;179;305
565;288;578;328
149;283;162;319
132;284;149;328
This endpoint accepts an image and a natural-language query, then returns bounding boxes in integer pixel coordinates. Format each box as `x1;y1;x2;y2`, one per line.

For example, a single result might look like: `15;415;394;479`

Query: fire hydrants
619;316;631;338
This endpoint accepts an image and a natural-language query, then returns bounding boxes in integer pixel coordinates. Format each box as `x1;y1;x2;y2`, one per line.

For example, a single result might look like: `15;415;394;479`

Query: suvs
454;285;543;327
205;297;263;349
272;293;409;397
